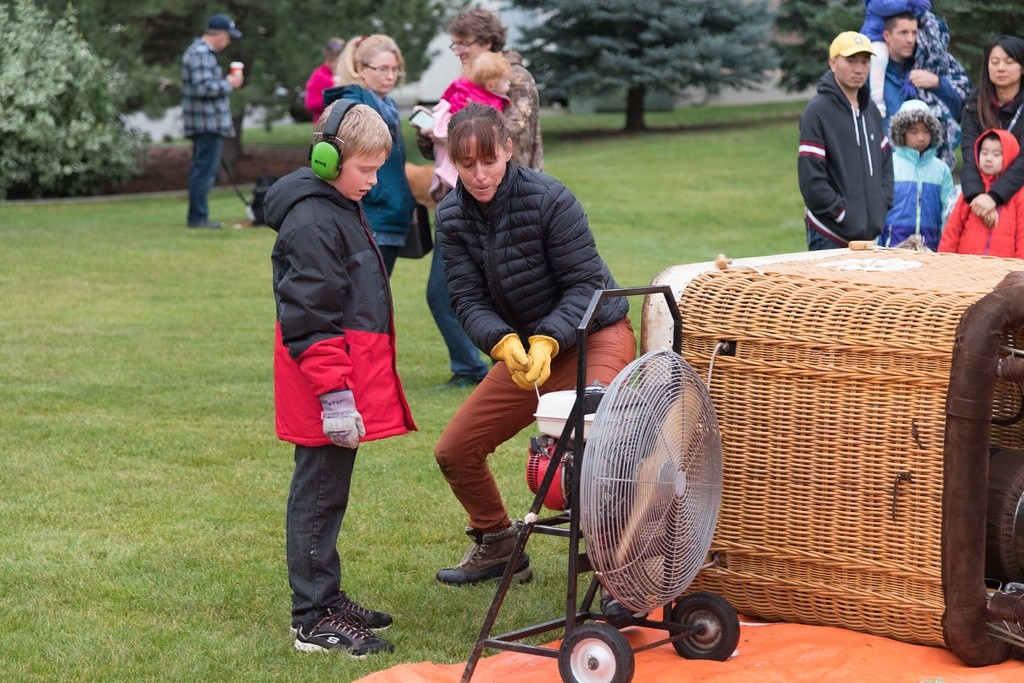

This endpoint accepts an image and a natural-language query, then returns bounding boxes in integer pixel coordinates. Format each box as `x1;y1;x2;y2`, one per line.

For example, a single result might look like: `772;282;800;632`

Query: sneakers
288;594;392;640
436;524;531;588
293;607;393;658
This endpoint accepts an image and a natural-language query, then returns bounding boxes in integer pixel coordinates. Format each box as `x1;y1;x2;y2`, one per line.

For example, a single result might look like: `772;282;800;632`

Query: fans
460;282;740;683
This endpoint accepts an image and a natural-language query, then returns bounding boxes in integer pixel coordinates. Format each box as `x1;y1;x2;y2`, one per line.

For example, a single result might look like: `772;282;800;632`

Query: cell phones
409;109;436;129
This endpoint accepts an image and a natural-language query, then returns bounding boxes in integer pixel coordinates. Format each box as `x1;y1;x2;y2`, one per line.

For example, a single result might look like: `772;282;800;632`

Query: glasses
359;62;402;75
451;36;482;52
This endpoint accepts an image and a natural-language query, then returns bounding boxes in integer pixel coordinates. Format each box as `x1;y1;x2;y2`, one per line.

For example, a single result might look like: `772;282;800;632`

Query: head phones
308;97;357;182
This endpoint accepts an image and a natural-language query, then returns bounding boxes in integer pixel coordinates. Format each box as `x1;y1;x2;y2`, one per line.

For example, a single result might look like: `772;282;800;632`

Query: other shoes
434;373;476;389
875;100;886;118
186;218;222;229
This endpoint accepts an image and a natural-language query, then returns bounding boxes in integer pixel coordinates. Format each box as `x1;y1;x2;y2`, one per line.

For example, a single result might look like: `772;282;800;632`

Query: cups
230;61;244;77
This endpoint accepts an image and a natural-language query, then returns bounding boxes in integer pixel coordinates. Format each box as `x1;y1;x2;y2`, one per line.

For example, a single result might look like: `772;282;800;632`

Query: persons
303;10;543;392
184;13;244;228
261;98;420;660
434;101;647;628
798;0;1024;264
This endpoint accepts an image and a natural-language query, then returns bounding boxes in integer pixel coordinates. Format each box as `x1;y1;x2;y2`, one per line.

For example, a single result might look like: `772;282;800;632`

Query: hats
208;15;243;39
828;32;875;61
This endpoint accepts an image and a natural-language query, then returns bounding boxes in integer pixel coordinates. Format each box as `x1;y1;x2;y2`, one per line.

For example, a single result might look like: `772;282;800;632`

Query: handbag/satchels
396;205;433;259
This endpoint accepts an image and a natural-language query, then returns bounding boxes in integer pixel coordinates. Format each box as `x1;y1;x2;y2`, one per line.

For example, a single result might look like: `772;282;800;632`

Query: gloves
318;388;366;449
512;335;561;390
490;334;533;375
